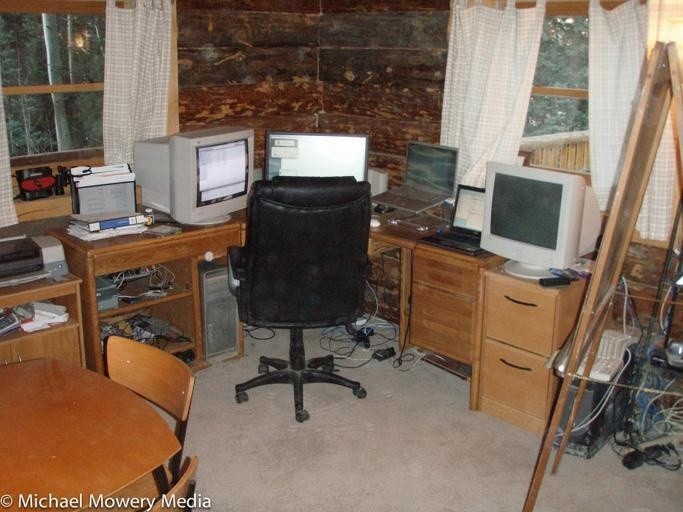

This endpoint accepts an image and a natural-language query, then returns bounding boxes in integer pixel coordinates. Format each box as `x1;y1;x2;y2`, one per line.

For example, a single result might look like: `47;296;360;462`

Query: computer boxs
197;261;240;364
554;344;636;459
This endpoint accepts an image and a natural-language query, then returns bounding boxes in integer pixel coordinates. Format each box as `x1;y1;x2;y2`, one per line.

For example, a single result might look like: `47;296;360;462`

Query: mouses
370;218;381;227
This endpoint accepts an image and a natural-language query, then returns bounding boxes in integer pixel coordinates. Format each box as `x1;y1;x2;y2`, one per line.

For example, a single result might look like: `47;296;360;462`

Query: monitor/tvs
263;131;369;182
133;124;256;225
479;161;602;281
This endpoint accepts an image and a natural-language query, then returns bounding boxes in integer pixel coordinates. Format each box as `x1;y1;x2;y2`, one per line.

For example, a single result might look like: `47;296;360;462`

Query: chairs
77;334;197;512
134;454;197;512
227;176;374;422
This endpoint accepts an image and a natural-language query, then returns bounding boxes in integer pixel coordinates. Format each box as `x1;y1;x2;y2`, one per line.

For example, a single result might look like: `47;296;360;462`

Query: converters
623;444;663;469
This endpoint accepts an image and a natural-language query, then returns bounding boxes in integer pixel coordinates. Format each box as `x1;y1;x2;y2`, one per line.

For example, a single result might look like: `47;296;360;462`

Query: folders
70;215;145;232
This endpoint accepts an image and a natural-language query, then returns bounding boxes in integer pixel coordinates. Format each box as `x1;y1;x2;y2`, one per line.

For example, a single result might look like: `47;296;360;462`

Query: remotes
540;277;572;290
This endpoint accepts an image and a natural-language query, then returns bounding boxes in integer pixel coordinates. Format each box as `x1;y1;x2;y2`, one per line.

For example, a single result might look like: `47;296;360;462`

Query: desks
1;356;183;512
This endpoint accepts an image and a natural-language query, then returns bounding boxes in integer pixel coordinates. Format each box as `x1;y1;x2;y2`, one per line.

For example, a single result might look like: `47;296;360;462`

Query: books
0;304;72;335
64;162;147;242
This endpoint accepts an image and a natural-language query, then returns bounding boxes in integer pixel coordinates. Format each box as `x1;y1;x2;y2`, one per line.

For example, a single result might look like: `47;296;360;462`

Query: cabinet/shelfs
230;189;451;353
411;239;509;367
1;273;87;370
470;269;588;441
44;219;244;376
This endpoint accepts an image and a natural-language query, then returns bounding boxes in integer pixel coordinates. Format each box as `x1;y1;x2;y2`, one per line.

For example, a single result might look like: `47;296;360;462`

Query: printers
0;234;68;288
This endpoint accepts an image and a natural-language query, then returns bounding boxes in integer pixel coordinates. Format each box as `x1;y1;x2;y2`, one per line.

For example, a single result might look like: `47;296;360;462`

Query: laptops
371;140;459;214
417;184;486;257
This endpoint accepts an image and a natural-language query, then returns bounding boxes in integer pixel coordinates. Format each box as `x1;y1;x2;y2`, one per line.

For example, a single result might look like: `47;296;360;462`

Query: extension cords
620;434;680;460
